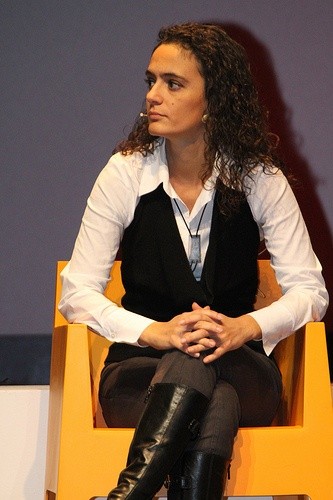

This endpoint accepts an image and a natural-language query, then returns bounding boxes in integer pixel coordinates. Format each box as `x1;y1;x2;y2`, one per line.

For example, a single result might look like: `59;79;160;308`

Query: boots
165;449;229;500
103;381;208;500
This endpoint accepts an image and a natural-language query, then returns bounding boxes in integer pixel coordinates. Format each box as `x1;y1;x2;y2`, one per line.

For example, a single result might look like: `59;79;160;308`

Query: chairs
44;259;333;500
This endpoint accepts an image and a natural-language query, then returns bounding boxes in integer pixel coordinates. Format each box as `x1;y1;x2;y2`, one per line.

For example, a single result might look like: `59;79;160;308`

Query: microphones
140;113;148;117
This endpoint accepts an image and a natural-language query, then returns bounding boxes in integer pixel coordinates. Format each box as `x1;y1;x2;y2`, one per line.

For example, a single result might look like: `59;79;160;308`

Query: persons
57;22;330;500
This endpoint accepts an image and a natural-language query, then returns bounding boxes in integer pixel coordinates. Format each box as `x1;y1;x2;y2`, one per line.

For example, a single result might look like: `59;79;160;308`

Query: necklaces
173;197;208;273
